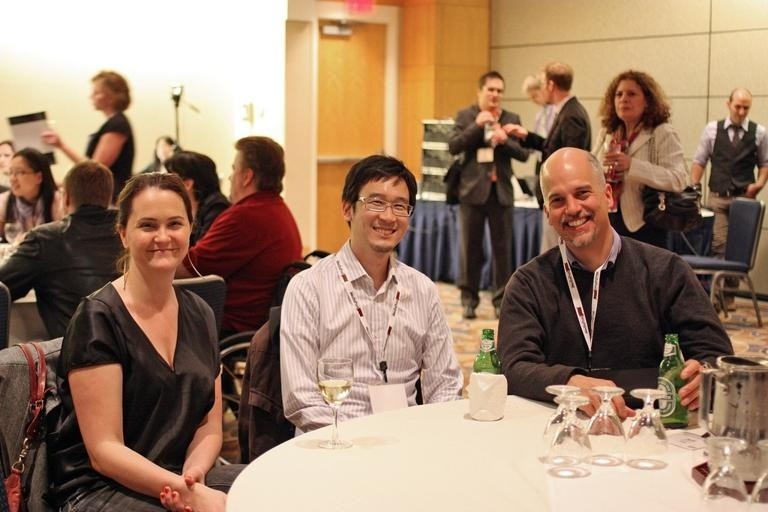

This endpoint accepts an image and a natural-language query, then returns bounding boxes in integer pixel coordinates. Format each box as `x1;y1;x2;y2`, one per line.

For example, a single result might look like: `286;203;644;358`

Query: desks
390;190;714;299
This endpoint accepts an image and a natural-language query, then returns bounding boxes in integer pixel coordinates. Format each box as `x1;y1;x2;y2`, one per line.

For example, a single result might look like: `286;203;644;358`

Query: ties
732;126;740;148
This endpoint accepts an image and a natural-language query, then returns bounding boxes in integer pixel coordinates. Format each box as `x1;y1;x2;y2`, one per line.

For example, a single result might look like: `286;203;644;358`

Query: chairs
681;196;766;329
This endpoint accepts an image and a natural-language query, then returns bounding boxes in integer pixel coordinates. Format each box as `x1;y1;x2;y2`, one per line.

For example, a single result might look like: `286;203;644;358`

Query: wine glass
317;359;354;451
698;437;746;511
602;143;622;185
747;439;768;509
537;383;674;480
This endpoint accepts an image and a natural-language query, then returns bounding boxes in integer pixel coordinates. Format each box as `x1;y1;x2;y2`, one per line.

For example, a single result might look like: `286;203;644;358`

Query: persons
496;146;734;422
39;71;135;207
591;69;690;252
690;88;768;315
43;173;248;511
502;60;591;255
0;135;302;414
521;73;559;138
448;70;530;320
280;154;465;438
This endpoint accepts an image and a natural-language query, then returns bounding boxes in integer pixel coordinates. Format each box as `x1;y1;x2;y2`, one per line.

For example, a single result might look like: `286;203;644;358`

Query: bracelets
697;360;712;370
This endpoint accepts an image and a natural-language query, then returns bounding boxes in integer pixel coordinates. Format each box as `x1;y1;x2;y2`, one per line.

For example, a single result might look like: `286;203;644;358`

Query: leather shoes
463;306;474;318
495;306;500;318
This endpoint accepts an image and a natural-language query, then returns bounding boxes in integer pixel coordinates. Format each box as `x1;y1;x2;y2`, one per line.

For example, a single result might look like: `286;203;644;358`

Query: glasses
359;197;413;217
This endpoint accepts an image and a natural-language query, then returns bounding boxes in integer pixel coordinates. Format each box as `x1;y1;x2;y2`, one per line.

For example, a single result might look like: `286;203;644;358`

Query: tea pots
699;356;767;444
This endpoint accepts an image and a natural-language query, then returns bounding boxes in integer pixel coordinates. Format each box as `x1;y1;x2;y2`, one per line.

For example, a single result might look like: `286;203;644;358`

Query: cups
3;223;22;245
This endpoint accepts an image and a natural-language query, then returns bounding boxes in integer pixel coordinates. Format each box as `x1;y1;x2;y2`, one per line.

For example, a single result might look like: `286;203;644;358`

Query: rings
614;161;618;166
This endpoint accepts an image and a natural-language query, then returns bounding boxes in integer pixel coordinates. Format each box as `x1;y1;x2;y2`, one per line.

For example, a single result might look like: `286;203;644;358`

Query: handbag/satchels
0;468;21;512
443;159;461;204
642;183;703;230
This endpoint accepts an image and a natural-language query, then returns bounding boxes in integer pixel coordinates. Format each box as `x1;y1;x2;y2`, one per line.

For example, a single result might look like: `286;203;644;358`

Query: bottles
658;332;693;432
468;327;506;422
